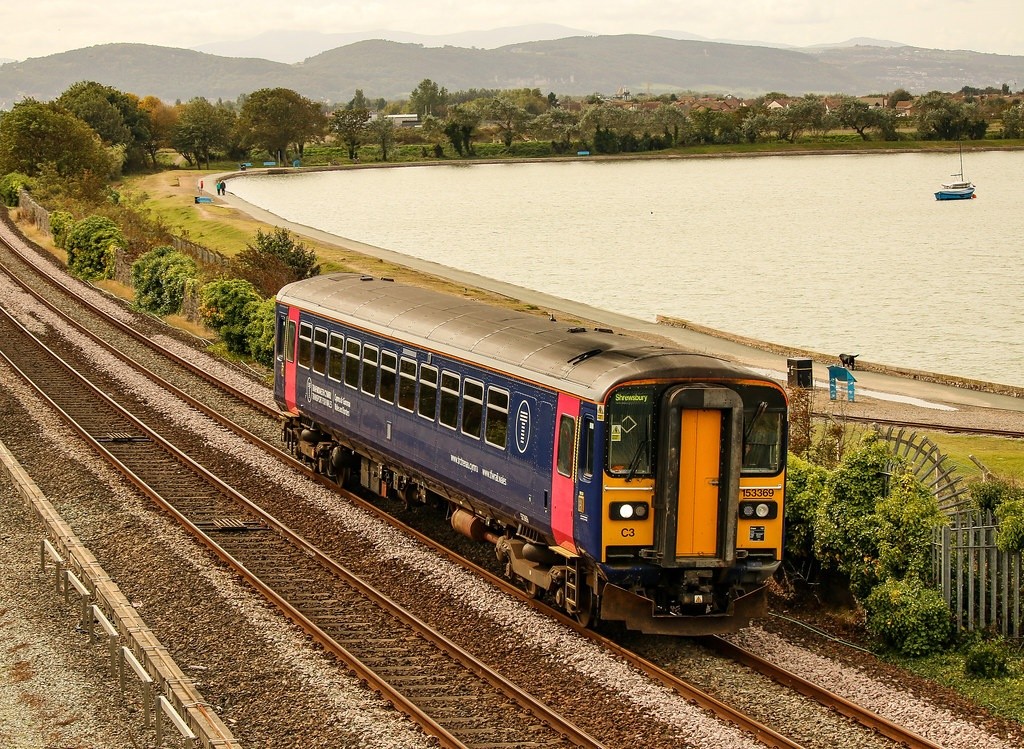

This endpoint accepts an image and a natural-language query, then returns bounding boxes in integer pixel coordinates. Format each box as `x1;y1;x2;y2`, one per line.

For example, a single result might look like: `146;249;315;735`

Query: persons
216;182;221;195
220;180;226;195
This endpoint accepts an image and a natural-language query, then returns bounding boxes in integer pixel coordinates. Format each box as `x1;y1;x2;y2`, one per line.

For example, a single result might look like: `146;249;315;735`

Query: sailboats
942;141;972;189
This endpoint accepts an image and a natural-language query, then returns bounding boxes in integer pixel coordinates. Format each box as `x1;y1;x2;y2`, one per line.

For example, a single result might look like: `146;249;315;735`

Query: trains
270;273;791;637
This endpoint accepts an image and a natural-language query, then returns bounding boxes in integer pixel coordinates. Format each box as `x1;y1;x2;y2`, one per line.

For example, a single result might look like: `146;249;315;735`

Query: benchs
242;163;253;168
264;162;276;167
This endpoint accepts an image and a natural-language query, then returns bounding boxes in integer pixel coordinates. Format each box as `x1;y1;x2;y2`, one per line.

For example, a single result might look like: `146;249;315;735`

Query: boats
934;187;976;200
